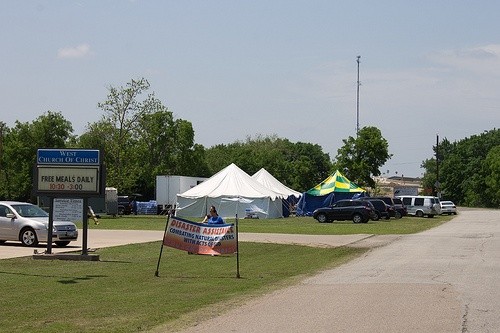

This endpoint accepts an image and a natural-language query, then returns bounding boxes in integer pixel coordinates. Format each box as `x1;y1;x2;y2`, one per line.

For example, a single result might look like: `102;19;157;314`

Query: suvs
361;197;396;216
369;200;389;221
393;198;407;218
394;196;441;218
312;199;376;223
118;195;132;215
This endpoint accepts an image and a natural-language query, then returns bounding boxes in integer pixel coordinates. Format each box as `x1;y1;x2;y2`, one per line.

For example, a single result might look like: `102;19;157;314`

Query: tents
251;167;303;217
175;163;282;219
296;169;366;217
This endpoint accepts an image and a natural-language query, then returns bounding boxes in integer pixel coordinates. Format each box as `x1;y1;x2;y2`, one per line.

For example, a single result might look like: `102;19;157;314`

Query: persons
208;210;223;224
202;205;218;223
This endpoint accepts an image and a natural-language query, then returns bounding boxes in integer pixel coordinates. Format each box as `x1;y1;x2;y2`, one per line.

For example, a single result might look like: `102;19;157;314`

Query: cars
440;201;458;215
0;201;78;247
